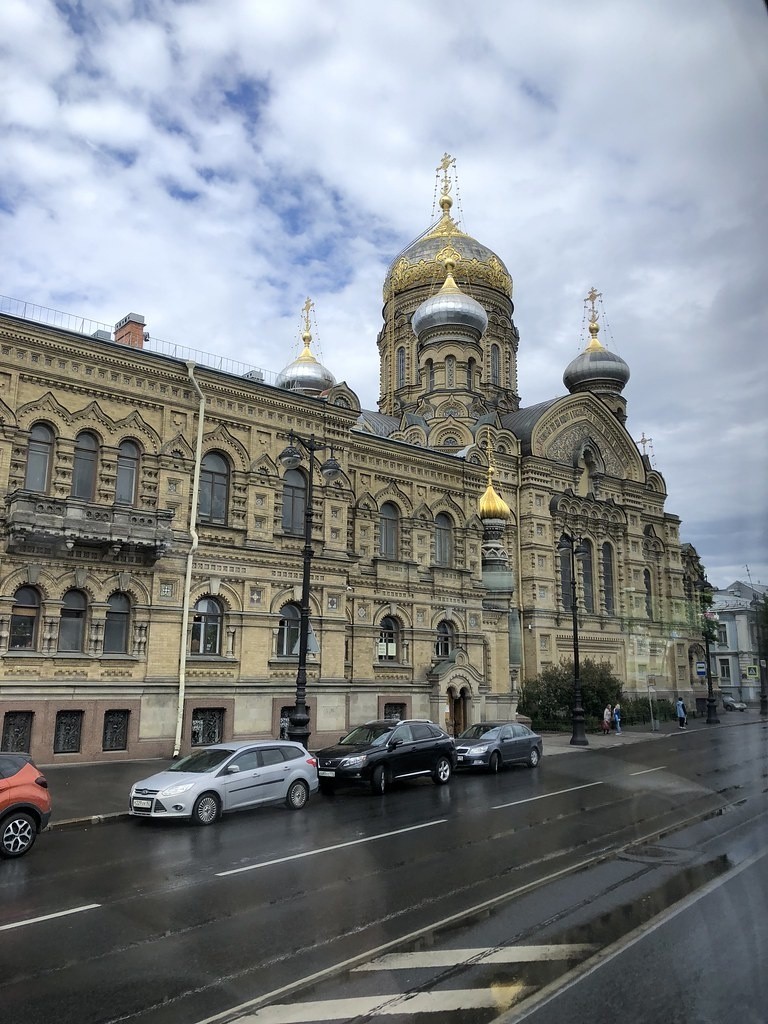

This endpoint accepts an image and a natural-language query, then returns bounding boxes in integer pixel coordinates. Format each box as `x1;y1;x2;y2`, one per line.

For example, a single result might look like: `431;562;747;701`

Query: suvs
313;719;459;797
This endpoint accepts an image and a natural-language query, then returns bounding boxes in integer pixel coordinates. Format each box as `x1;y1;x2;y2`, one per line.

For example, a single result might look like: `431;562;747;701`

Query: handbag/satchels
601;723;610;729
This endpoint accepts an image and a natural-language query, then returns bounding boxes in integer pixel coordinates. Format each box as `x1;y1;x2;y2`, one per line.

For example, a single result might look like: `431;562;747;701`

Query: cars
127;738;321;827
1;750;53;859
723;696;747;712
453;719;544;776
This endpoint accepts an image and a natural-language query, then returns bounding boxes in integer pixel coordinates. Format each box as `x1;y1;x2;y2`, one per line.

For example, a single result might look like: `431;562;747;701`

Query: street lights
558;521;595;746
279;426;344;753
700;617;721;724
749;592;768;714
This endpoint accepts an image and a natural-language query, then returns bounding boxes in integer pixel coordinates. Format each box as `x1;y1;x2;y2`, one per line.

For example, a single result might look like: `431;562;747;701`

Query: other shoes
679;727;686;729
616;733;622;735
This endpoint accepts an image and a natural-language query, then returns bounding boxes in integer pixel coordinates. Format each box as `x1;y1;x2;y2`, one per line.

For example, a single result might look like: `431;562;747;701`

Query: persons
676;697;688;730
602;704;611;736
613;704;622;736
